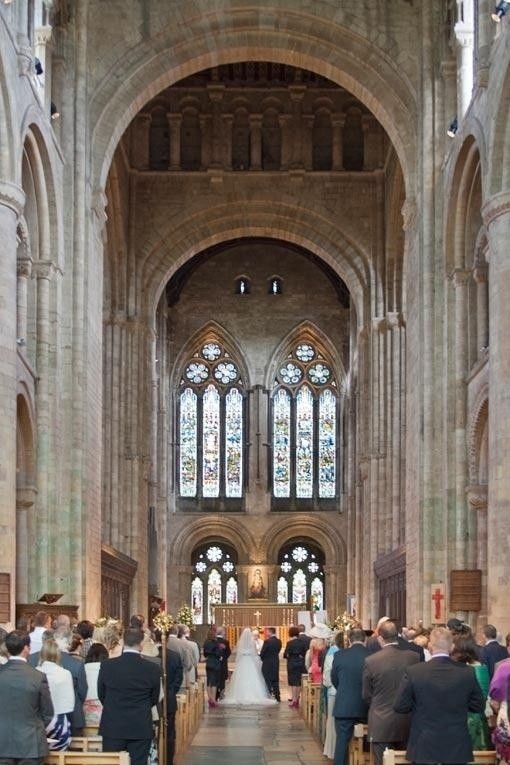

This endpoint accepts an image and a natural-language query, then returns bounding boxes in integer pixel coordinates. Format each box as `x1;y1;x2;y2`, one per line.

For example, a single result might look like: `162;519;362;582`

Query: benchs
45;676;210;765
300;675;509;765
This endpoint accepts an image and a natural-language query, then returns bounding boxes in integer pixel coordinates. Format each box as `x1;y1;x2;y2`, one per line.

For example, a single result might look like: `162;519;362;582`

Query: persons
215;626;231;701
0;610;201;765
203;624;224;707
251;629;264;656
259;627;283;702
218;626;278;706
283;616;510;765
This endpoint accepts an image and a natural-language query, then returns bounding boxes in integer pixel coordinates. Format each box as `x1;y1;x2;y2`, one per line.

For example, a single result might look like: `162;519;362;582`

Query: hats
308;624;332;638
448;619;463;631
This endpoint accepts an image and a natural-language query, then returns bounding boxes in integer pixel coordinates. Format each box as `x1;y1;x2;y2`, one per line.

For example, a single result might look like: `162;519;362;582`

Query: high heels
288;699;299;707
208;699;218;707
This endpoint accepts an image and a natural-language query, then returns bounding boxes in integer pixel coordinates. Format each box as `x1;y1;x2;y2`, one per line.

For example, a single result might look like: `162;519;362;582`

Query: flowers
176;603;196;632
152;611;174;633
